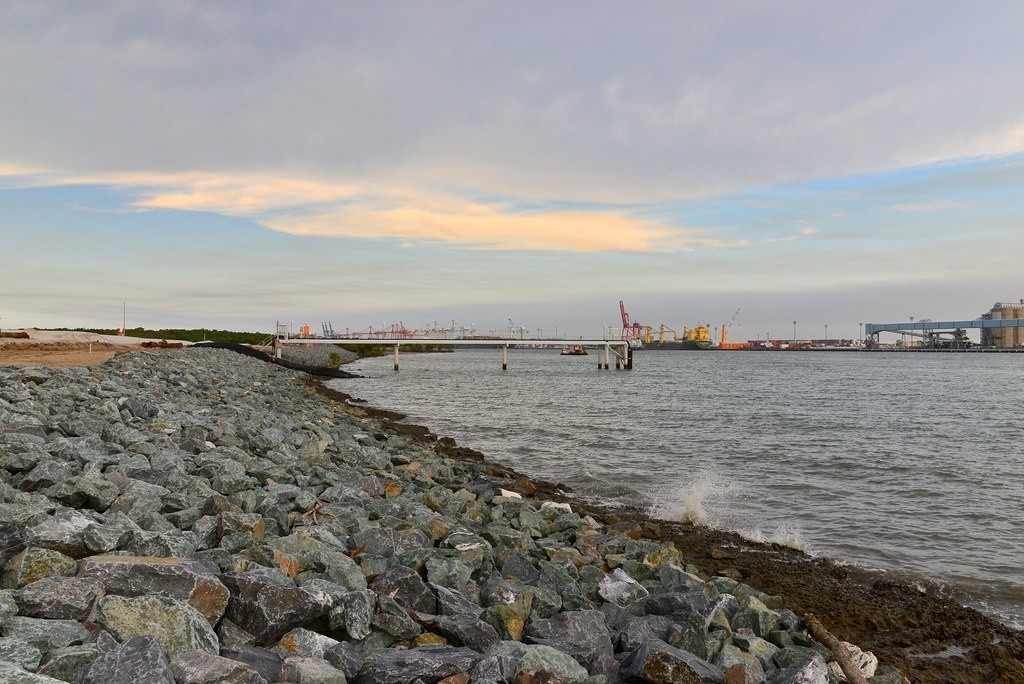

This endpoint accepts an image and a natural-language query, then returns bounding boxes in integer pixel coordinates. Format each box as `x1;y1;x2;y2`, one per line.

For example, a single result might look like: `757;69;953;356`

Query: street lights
824;324;828;346
910;316;914;348
859;322;862;348
793;320;796;341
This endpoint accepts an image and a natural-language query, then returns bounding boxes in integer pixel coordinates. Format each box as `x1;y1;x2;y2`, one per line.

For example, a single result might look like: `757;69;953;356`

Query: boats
560;349;588;355
